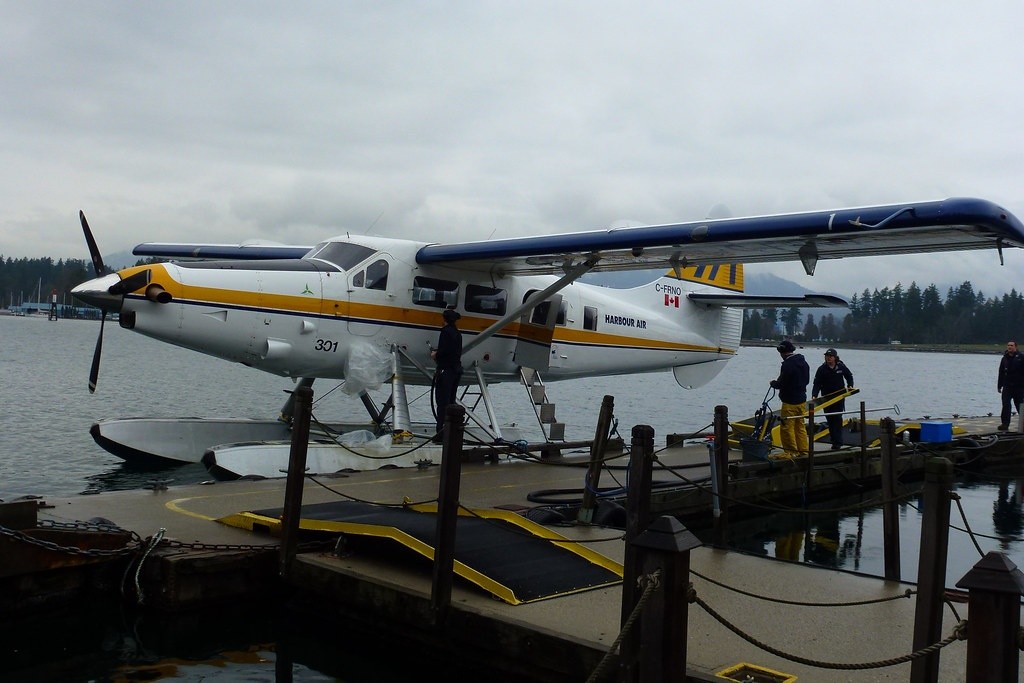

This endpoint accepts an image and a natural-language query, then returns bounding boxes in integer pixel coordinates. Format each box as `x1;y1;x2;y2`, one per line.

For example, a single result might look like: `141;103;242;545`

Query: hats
824;349;837;356
443;310;461;321
777;341;795;352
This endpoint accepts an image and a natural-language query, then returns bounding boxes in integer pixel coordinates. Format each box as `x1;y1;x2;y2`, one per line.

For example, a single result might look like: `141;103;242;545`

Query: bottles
426;340;438;364
903;429;910;442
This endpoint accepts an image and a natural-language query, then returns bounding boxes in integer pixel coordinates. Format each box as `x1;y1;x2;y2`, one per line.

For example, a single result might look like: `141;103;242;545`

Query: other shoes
998;423;1009;430
831;443;842;449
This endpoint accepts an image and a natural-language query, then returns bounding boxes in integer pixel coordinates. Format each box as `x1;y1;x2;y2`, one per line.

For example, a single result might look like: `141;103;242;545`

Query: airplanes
76;194;1024;483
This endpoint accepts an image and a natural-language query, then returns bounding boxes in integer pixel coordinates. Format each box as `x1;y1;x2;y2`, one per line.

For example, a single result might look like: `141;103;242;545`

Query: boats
7;277;102;320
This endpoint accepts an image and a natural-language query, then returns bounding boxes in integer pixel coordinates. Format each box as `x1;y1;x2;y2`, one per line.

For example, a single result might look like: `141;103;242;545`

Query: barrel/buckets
740;438;770;464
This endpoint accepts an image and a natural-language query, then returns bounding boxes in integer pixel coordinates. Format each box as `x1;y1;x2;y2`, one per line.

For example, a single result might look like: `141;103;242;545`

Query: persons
431;310;464;444
770;340;810;458
997;341;1024;432
811;348;855;452
772;514;857;569
